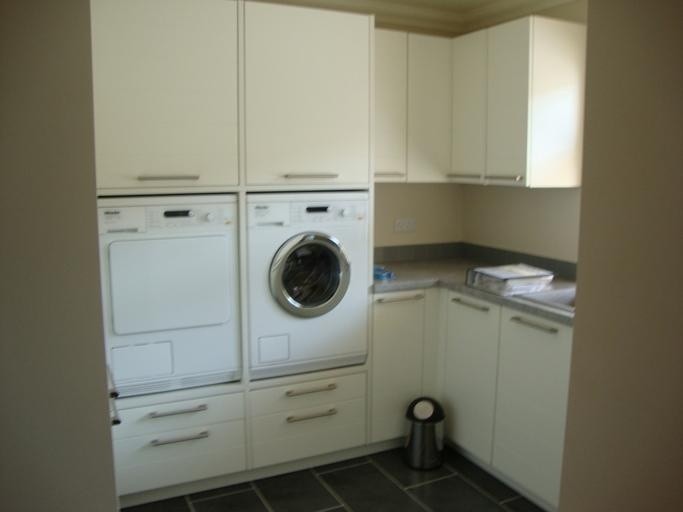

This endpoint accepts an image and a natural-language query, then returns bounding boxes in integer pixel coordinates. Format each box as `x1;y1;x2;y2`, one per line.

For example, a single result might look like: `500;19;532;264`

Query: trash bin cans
405;396;445;470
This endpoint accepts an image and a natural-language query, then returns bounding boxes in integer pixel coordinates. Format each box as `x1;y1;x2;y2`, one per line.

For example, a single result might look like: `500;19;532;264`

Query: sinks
512;285;576;314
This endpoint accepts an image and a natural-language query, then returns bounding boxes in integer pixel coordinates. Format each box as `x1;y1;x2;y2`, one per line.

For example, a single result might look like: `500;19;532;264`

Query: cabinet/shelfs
108;383;247;511
438;288;572;507
368;287;436;453
248;370;370;484
445;14;586;190
371;27;449;186
242;2;373;193
90;0;240;195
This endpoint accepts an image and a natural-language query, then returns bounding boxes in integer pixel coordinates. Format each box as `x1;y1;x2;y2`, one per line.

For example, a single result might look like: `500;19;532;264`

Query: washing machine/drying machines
245;191;368;382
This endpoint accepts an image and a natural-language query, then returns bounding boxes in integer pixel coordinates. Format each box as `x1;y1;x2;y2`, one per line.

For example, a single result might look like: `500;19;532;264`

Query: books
464;260;556;299
